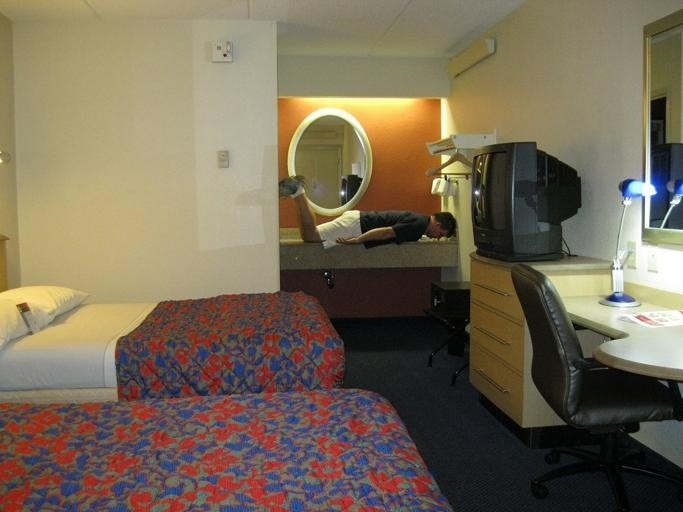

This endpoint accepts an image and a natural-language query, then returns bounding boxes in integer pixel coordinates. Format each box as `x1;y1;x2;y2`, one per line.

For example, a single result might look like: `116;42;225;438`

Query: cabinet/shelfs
468;249;613;449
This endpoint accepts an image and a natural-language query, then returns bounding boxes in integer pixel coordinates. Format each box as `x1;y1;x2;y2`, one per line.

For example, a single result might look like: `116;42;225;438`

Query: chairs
509;262;683;511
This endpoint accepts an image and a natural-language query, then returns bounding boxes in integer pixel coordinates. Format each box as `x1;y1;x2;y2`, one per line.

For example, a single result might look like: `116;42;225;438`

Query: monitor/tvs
650;141;683;228
340;174;361;204
473;142;582;263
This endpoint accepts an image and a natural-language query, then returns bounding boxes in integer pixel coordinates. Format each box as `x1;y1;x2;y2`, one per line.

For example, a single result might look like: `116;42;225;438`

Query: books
16;302;41;335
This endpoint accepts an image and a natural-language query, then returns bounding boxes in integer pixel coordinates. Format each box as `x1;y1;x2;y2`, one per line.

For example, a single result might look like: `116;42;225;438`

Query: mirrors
285;107;373;217
641;10;682;244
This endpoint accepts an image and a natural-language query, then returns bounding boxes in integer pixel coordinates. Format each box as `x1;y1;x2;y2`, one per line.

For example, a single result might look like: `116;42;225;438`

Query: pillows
1;284;87;347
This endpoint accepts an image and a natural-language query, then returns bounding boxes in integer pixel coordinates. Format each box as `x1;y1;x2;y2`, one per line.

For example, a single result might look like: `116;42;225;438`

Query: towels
430;178;456;198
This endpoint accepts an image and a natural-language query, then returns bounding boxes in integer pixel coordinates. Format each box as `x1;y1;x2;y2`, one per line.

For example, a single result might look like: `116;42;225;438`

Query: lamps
598;178;657;309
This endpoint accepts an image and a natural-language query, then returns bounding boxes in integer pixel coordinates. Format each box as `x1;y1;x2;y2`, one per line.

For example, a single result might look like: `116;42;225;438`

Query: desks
556;294;683;381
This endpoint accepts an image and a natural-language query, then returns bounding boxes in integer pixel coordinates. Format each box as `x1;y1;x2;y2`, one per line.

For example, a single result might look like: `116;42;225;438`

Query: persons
278;174;458;249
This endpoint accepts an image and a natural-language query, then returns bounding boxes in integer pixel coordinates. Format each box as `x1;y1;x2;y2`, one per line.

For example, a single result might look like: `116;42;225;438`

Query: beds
1;288;346;389
1;399;454;512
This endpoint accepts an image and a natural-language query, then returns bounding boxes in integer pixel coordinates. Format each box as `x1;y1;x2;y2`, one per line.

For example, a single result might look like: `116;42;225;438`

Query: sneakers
279;175;305;200
